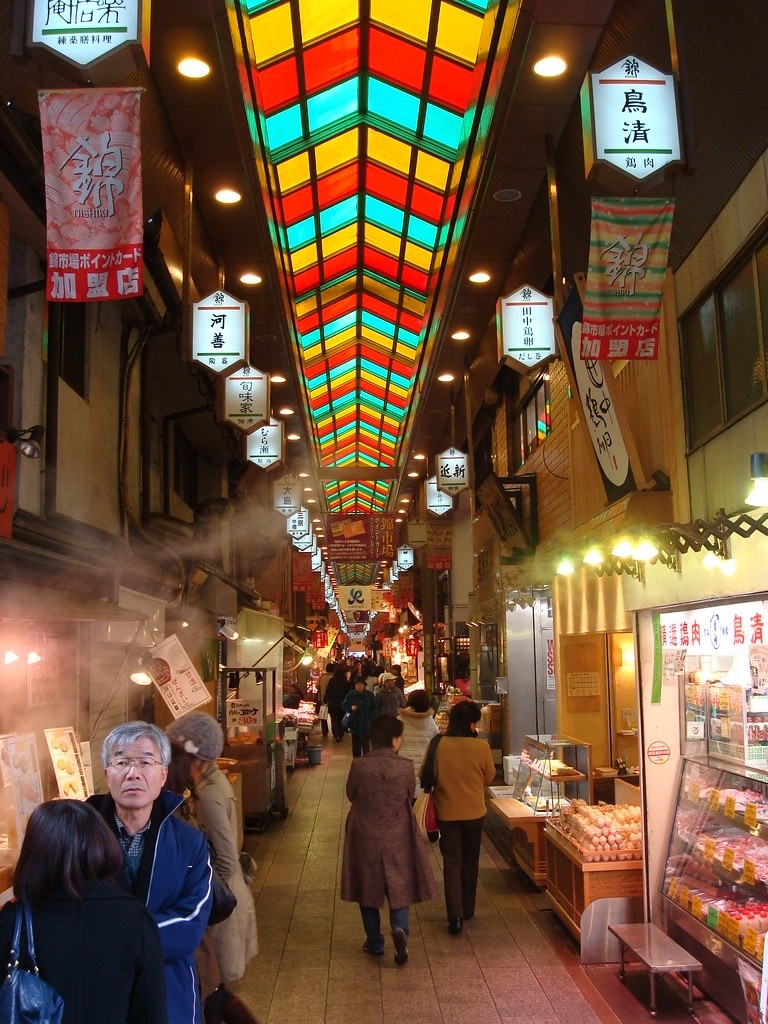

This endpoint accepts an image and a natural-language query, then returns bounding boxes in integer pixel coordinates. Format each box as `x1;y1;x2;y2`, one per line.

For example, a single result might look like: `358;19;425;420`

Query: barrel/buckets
307;745;322;765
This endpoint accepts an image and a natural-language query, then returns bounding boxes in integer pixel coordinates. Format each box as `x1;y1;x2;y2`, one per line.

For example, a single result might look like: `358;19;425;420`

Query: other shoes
363;942;385;955
448;918;463;934
336;736;340;742
392;929;408;965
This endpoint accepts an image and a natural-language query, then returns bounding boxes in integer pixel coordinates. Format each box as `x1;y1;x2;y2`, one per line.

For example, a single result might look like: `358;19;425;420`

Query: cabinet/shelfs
660;755;768;1024
490;733;594;887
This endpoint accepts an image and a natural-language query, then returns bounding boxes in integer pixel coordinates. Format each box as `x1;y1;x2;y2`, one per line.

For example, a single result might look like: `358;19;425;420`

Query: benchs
608;922;702;1017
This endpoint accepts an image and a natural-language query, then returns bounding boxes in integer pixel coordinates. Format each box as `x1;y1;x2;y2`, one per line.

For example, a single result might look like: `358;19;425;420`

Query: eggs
684;851;720;886
568;806;642;862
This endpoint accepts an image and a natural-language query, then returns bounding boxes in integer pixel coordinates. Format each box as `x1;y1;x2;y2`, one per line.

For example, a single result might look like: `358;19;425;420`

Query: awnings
0;580;146;736
236;606;316;677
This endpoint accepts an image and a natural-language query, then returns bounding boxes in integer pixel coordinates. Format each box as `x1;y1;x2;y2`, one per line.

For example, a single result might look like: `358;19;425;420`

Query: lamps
218;619;239;640
745;452;768;507
130;652;153;685
226;673;238;691
254;672;264;686
6;425;44;459
465;611;488;627
502;585;536;611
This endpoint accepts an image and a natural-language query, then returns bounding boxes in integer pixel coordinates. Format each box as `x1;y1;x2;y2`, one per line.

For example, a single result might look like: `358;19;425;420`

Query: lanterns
272;471;302;519
190;288;250;380
25;0;150;82
285;505;348;634
388;544;414;585
436;447;469;497
221;367;270;434
580;56;694;195
424;475;456;517
243;416;286;472
496;285;561;375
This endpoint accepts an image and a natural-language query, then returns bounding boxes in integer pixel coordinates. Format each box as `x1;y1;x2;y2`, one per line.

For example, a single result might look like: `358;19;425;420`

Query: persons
85;722;214;1024
340;717;438;966
418;701;496;935
283;683;304;710
167;712;260;1024
316;655;406;757
0;799;168;1024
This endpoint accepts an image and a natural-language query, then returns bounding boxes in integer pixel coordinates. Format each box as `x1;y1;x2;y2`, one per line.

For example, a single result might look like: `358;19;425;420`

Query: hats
352;676;367;686
381;673;397;685
168;712;224;762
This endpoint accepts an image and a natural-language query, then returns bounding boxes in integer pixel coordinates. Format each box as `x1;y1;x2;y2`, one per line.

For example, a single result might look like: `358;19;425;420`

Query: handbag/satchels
319;704;328;720
341;712;351;727
0;904;64;1024
412;789;439;843
207;867;237;926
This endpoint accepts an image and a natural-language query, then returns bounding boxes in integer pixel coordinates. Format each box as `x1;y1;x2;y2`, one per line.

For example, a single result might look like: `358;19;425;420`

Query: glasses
106;757;163;773
